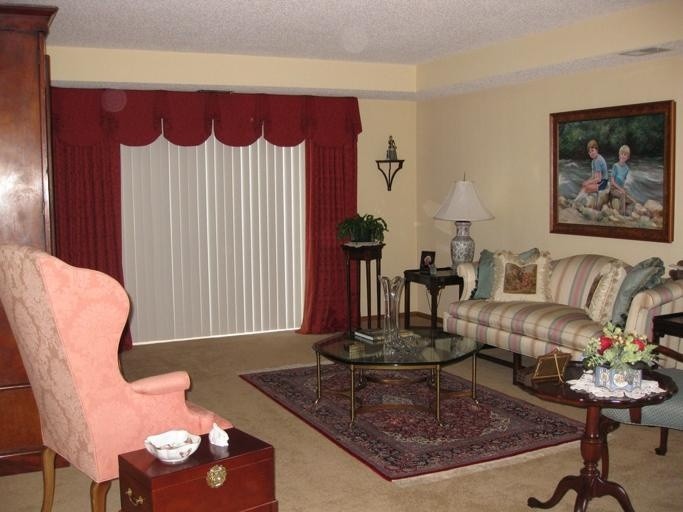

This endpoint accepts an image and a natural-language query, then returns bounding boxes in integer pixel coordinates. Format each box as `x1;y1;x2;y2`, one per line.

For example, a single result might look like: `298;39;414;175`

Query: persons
581;139;608;193
609;144;632;216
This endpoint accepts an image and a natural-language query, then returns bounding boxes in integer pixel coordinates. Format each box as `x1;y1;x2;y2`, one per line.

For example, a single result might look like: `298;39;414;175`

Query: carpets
238;363;586;482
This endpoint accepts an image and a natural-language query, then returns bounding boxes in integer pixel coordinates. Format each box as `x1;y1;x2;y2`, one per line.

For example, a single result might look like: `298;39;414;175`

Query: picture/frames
549;101;677;244
532;349;571;386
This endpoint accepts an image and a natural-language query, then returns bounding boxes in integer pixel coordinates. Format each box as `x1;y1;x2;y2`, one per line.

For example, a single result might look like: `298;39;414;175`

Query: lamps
432;180;496;268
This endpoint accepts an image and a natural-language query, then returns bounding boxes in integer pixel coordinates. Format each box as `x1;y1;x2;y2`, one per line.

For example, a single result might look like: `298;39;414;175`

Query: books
354;327;413;341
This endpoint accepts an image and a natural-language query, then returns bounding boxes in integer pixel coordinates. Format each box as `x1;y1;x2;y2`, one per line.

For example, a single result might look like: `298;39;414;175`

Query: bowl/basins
143;429;201;463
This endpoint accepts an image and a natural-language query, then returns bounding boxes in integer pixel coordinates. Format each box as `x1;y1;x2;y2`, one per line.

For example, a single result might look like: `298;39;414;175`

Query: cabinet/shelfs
118;426;277;511
0;5;70;477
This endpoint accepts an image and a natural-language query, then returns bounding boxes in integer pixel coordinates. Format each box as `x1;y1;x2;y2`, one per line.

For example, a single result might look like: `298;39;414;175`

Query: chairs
598;368;682;481
0;243;236;511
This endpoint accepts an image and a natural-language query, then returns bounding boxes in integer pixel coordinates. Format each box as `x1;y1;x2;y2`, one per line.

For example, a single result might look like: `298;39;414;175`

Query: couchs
442;255;683;386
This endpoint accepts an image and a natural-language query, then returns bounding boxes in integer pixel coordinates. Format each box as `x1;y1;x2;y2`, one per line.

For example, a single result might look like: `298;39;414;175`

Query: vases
595;366;642;391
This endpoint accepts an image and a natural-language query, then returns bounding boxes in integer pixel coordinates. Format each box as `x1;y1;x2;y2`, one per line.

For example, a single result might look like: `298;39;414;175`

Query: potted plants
336;215;390;246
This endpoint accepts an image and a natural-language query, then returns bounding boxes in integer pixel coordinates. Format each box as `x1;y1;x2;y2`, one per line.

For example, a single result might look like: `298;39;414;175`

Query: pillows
611;257;666;331
490;249;552;303
584;260;626;322
469;247;539;300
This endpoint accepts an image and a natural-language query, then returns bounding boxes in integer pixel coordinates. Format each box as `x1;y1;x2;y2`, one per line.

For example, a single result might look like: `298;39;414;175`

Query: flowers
583;321;665;369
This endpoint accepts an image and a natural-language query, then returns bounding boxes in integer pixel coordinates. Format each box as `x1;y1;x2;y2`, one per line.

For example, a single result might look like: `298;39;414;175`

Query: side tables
520;362;679;512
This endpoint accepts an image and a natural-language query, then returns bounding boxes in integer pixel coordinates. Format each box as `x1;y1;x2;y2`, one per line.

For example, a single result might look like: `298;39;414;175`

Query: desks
341;245;386;333
652;312;683;370
404;267;464;329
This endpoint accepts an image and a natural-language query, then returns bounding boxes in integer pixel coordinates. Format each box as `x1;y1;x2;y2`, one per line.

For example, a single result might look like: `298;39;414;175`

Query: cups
430;264;437;275
